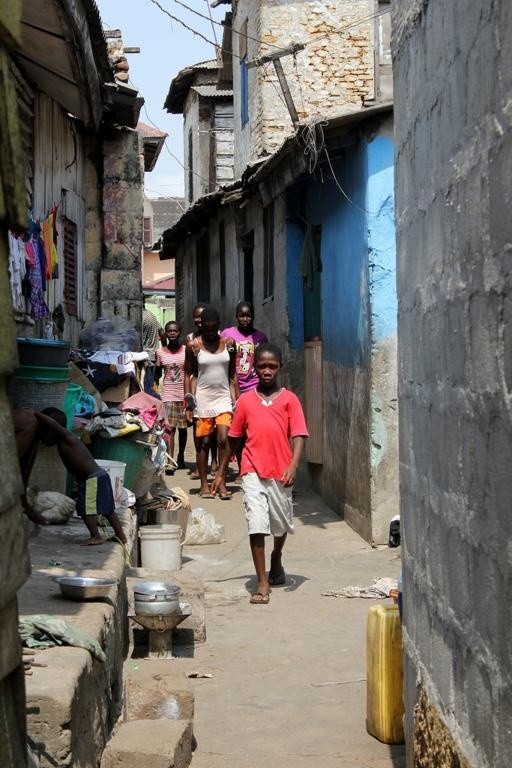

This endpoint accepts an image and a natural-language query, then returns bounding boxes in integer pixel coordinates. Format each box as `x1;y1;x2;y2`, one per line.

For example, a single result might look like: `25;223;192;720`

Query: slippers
166;455;242;499
267;565;286;585
251;588;270;604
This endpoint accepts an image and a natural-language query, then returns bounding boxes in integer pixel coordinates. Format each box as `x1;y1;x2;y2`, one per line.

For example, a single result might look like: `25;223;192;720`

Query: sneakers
388;515;400;547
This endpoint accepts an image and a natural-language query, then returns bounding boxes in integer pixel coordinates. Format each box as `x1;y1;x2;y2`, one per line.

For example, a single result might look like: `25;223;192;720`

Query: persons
210;342;311;605
141;292;270;500
34;406;129;546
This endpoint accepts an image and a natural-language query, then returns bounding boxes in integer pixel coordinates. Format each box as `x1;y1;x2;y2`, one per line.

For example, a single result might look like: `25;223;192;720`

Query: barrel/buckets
137;524;184;570
365;603;406;744
8;335;148;509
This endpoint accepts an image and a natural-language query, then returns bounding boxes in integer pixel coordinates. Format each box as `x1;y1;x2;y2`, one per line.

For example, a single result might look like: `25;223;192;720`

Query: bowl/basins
12;335;72;380
130;582;182;617
52;575;121;602
